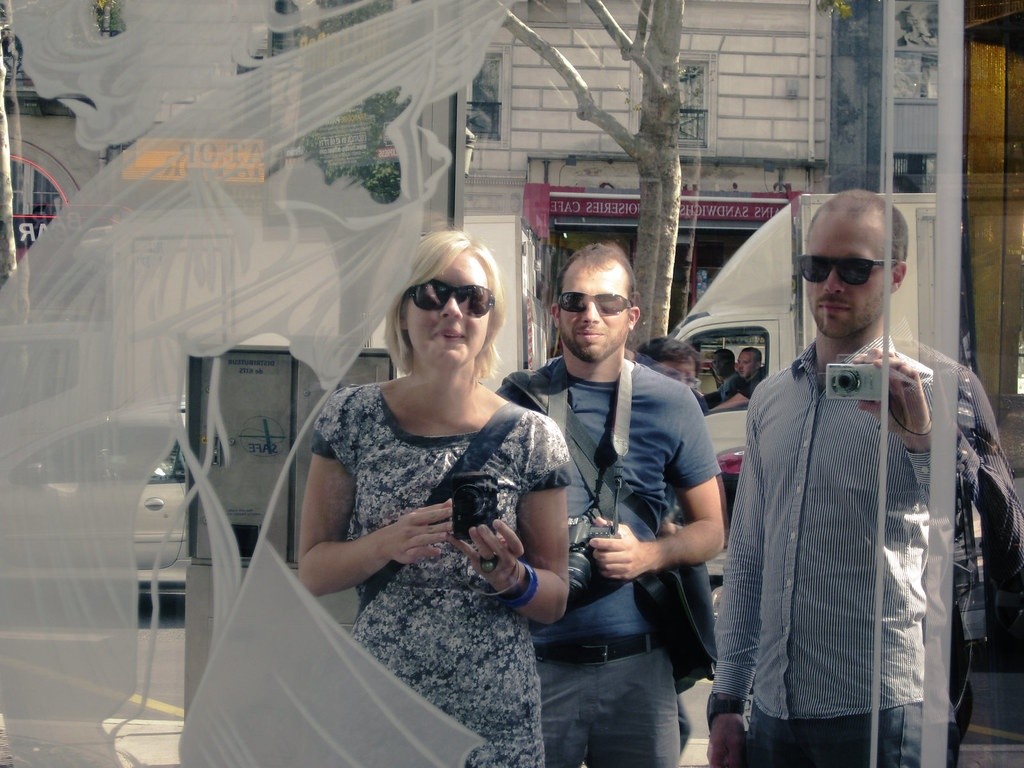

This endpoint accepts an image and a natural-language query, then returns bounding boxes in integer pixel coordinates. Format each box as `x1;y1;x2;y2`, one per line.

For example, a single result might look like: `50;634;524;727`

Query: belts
535;631;658;665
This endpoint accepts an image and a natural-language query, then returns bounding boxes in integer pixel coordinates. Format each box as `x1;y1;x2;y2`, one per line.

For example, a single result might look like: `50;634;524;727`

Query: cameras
825;358;897;402
452;472;504;541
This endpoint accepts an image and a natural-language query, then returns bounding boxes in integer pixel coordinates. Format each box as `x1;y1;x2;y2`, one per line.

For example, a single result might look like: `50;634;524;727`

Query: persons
628;332;705;418
855;227;1024;768
711;338;763;419
470;238;730;768
707;348;746;413
702;188;951;768
292;226;575;768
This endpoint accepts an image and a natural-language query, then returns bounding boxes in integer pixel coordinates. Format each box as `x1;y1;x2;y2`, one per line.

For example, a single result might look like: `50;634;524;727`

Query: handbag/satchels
632;479;717;683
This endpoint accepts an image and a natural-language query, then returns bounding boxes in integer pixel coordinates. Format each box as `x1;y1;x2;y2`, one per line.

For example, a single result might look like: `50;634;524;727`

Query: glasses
795;254;898;286
406;279;495;318
682;372;702;387
557;291;633;315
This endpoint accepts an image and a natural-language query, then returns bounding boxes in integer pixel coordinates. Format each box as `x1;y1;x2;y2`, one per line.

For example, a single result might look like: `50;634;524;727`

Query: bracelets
498;562;542;610
463;557;523;597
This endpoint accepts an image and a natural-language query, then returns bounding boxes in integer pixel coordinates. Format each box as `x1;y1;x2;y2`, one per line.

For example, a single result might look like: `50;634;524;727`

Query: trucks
116;328;402;609
653;193;948;506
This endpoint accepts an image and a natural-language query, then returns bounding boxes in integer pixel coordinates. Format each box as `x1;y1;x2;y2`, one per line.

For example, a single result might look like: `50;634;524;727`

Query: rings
478;551;500;574
